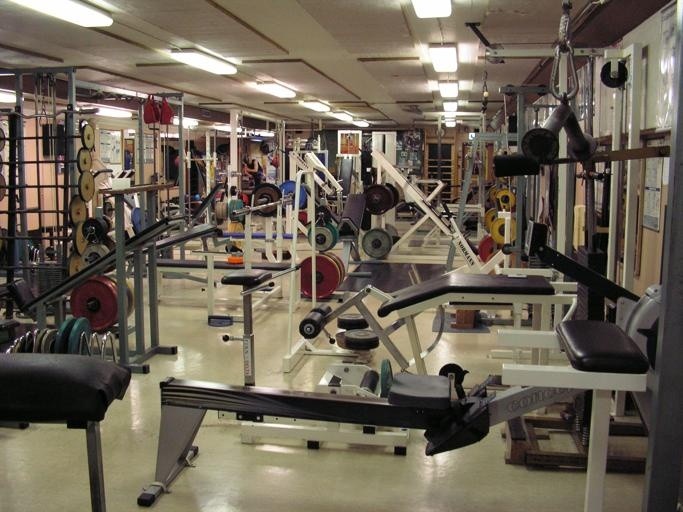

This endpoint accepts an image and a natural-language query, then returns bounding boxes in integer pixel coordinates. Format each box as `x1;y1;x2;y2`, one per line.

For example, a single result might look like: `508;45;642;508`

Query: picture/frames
335;129;362;158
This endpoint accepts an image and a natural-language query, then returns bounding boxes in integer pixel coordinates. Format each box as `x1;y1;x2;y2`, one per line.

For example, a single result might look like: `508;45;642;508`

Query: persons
241;152;263;185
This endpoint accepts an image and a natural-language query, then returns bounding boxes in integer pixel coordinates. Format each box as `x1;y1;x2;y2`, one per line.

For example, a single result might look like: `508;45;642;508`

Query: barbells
0;124;94;291
